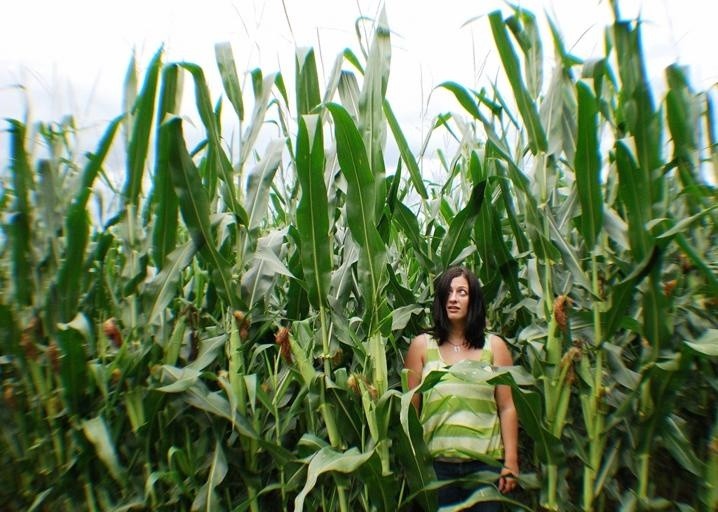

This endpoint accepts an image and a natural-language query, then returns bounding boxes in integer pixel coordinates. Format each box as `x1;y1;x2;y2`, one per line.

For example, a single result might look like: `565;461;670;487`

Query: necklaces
446;338;468;352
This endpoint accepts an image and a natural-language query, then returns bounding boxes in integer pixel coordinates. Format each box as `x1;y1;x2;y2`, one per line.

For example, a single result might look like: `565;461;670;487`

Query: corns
1;250;704;449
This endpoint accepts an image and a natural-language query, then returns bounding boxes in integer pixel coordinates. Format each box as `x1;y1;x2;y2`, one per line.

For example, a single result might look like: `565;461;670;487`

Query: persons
404;267;519;512
